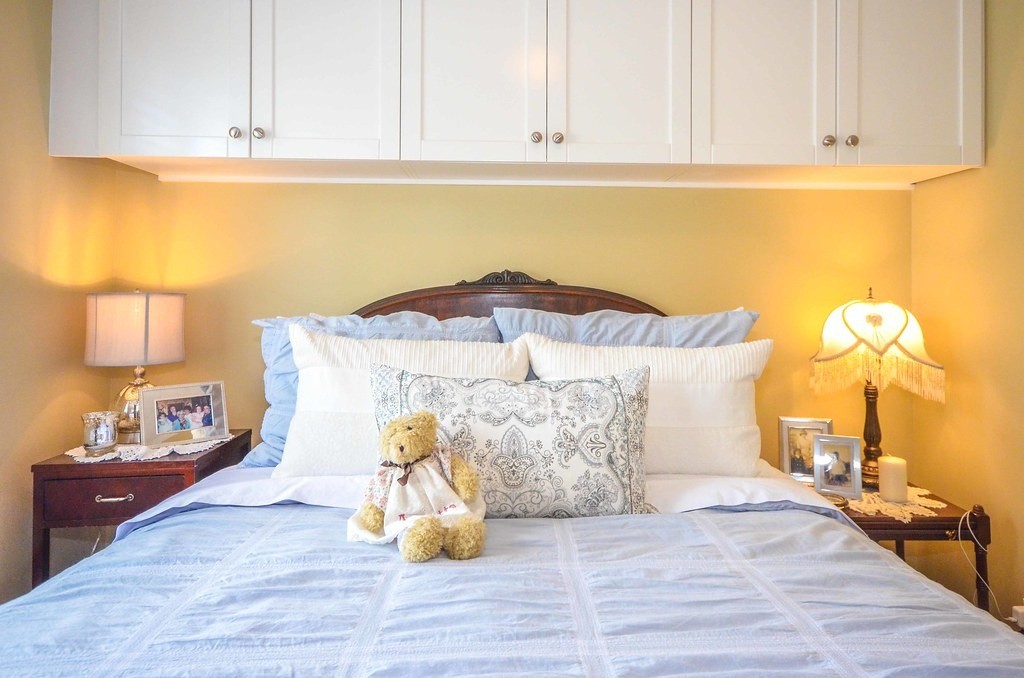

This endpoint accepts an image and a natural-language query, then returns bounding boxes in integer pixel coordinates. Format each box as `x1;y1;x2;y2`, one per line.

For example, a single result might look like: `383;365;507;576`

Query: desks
842;482;991;617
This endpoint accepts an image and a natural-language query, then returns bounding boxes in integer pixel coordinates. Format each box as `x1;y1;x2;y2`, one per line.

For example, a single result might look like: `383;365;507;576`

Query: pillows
242;312;503;467
525;331;774;478
362;363;661;518
493;307;760;382
271;323;529;478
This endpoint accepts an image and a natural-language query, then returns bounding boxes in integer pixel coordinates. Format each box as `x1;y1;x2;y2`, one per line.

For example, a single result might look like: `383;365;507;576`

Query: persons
157;402;213;433
793;430;849;485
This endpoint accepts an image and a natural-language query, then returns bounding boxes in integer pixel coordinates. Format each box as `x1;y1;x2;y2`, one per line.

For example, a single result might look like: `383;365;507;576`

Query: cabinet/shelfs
51;0;986;189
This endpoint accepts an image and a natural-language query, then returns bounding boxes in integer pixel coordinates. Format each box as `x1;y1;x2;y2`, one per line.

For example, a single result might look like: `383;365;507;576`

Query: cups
80;411;121;459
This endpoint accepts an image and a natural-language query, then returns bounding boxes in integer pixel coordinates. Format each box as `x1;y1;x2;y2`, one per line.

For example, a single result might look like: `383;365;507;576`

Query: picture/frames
812;434;862;501
138;380;229;448
779;416;833;483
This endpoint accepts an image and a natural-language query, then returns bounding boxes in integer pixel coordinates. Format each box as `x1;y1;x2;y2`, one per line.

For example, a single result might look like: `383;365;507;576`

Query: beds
0;267;1024;678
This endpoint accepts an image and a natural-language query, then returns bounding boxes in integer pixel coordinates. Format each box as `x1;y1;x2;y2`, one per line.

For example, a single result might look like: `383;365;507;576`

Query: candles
876;452;908;502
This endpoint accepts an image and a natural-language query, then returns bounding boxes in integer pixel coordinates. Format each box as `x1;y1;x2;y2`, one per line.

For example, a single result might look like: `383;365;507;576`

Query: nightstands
31;429;250;589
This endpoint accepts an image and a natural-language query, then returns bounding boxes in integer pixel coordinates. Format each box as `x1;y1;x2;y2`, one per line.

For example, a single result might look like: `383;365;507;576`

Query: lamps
806;286;945;488
83;288;186;444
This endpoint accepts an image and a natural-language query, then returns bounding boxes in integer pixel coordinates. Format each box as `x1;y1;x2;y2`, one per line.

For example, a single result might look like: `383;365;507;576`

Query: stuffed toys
360;412;485;561
